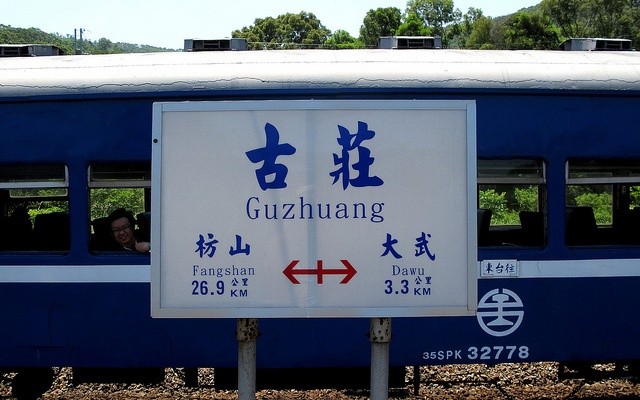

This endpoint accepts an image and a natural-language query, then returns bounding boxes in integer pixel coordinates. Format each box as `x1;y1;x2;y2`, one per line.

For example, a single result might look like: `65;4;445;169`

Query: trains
0;35;640;388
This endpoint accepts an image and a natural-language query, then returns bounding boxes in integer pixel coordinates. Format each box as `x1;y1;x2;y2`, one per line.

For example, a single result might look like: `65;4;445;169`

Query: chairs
0;216;33;251
565;206;598;246
35;211;71;251
133;211;151;242
519;210;544;243
92;216;109;251
478;208;493;232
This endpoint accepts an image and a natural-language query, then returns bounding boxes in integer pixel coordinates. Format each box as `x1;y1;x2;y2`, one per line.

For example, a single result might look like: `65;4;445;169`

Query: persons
108;208;150;253
10;205;33;250
